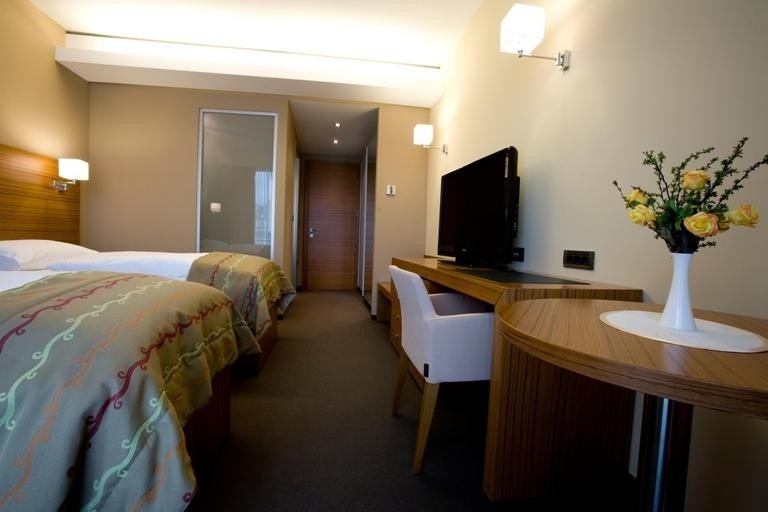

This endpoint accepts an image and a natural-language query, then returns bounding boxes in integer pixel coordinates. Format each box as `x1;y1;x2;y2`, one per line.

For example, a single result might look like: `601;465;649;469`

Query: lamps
499;3;571;71
413;124;448;154
53;158;89;192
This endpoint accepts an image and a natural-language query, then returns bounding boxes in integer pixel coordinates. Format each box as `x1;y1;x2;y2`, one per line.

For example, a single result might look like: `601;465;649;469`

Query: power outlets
563;250;595;271
512;248;525;262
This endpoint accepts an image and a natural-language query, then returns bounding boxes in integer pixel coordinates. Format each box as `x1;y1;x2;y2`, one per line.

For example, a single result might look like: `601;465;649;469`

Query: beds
1;270;262;512
0;238;297;376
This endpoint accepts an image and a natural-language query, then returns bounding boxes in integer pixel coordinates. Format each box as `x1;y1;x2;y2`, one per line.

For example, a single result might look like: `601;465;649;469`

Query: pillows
0;239;100;271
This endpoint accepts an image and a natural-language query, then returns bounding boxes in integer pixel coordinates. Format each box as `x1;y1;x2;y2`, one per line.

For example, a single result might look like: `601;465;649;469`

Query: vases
658;253;700;332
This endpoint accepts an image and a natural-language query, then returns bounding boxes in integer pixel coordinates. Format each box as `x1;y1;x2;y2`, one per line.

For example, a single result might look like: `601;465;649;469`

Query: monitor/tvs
436;146;521;270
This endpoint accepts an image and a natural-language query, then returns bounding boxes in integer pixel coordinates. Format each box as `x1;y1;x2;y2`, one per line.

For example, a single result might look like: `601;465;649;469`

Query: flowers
612;136;768;254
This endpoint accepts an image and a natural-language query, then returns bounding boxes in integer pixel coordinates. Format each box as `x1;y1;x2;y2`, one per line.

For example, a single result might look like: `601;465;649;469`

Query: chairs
389;264;497;478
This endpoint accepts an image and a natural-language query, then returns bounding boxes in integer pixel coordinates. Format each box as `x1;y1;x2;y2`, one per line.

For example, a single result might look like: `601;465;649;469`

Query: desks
377;282;391;328
496;298;767;512
390;255;645;505
